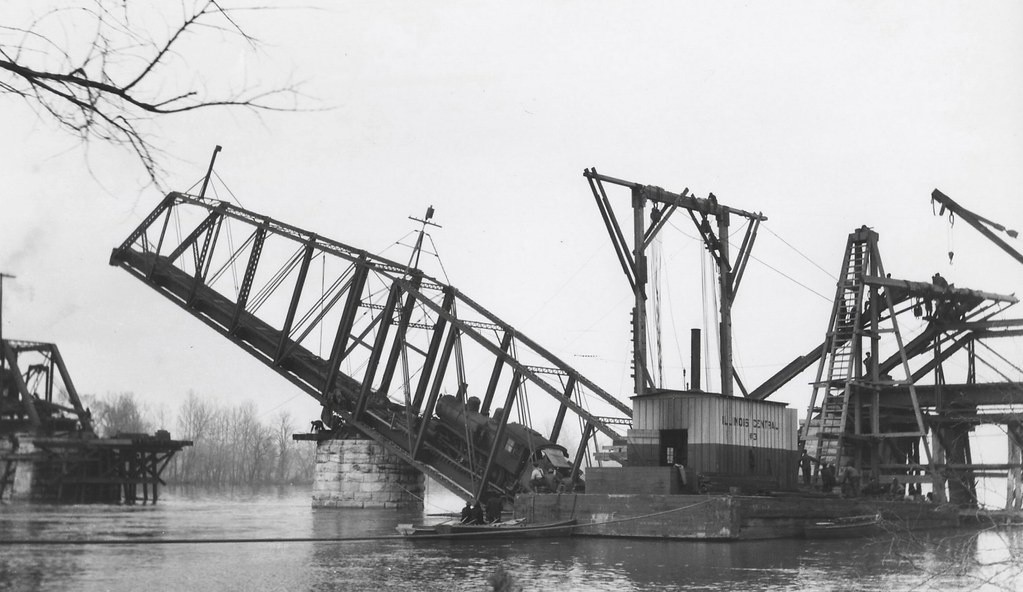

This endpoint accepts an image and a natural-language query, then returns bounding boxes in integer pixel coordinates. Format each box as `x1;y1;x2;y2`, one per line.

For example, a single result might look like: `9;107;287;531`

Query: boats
394;516;578;540
805;510;882;541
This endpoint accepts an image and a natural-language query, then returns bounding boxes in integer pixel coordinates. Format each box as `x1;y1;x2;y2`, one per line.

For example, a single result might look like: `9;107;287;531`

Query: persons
842;465;860;499
863;352;871;373
486;493;504;523
748;446;758;474
799;451;816;486
310;420;325;433
890;477;905;501
469;501;485;525
708;192;717;208
85;406;91;421
333;415;343;425
923;297;933;316
461;500;472;522
821;461;836;493
935;273;948;287
546;468;562;493
861;476;885;500
529;461;546;494
